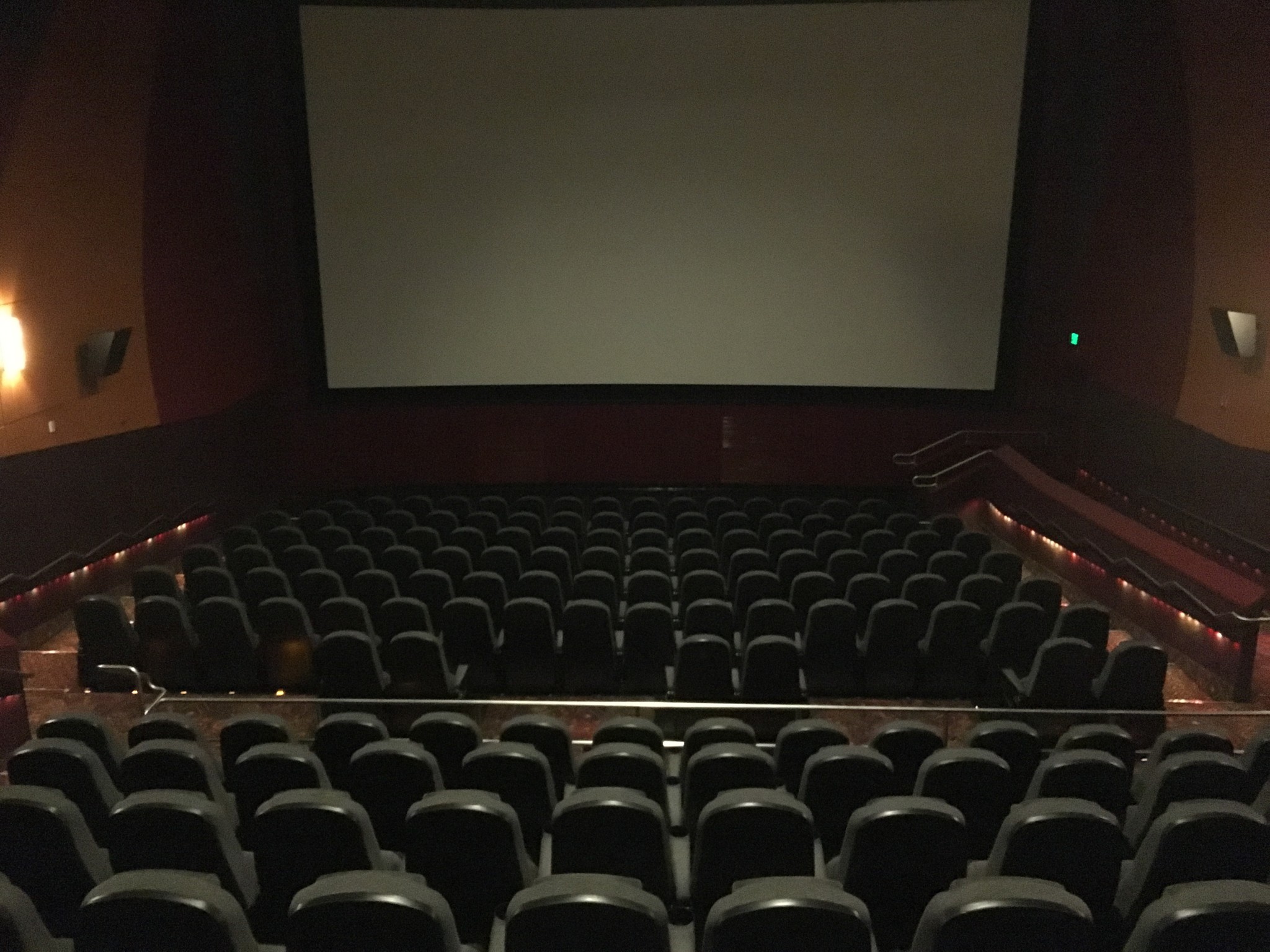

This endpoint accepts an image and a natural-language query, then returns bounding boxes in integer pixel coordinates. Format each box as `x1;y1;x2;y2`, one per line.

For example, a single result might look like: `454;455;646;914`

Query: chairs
0;483;1270;952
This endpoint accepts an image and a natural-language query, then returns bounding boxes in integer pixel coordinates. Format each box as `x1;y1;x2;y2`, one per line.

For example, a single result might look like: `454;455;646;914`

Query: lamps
79;328;134;380
1213;309;1260;359
